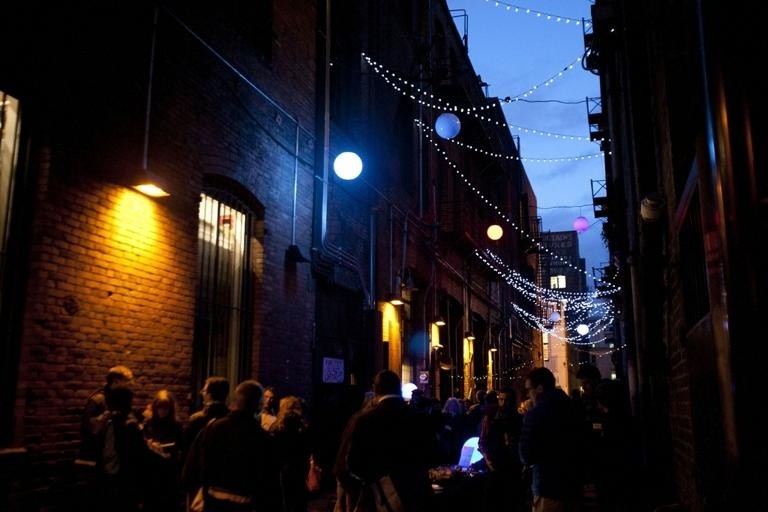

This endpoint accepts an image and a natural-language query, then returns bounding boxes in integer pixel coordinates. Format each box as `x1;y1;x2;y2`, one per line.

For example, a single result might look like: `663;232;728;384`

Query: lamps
131;170;175;200
386;270;502;353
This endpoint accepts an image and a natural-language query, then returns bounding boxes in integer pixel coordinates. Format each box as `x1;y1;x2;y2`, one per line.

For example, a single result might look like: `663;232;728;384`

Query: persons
335;365;653;509
84;366;310;510
